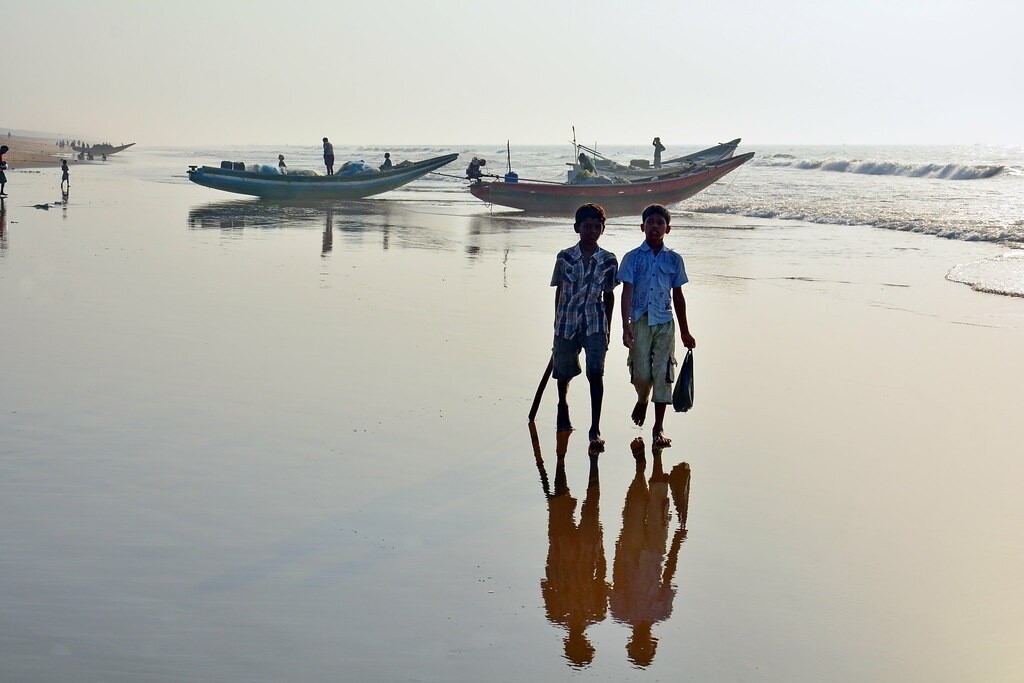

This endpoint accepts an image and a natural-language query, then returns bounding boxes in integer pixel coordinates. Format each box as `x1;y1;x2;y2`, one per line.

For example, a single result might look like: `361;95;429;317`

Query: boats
74;142;136;155
187;153;460;202
471;151;755;214
567;138;740;184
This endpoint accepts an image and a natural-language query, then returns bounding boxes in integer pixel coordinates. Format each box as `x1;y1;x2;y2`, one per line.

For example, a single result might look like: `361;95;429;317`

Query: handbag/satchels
672;349;694;413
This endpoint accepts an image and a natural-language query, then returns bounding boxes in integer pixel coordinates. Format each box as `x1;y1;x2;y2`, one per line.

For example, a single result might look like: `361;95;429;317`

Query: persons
652;136;666;169
606;435;693;669
549;203;620;446
60;160;71;188
577;153;598;175
322;137;335;176
0;145;9;196
538;428;610;669
379;152;393;171
54;137;125;162
616;204;696;446
278;154;287;169
